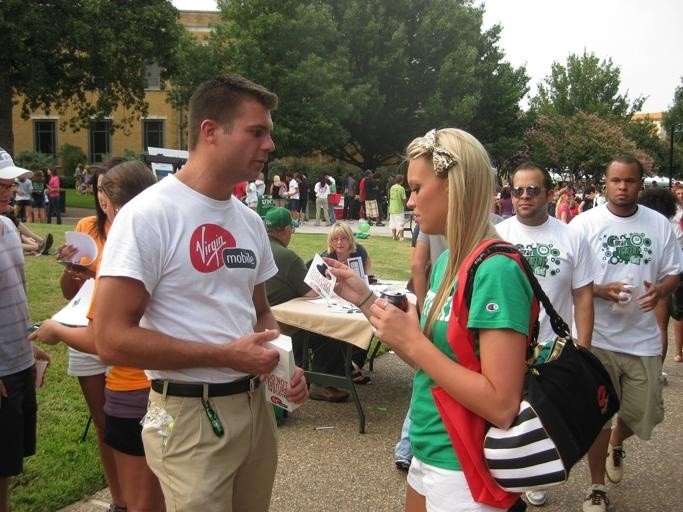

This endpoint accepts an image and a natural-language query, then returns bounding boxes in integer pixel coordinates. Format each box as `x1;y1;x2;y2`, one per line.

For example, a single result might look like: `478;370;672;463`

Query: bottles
609;270;639;316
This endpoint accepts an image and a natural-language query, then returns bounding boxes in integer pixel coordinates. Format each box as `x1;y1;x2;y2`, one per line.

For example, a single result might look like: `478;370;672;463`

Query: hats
0;147;35;180
264;206;299;231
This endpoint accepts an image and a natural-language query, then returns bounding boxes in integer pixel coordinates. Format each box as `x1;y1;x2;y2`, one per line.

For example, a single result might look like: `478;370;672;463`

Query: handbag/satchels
483;337;620;494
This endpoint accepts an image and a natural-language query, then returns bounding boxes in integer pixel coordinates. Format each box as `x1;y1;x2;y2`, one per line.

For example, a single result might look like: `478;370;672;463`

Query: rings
382;301;390;309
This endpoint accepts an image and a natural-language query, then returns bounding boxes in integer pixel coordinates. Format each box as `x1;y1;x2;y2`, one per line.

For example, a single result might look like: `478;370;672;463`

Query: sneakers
606;443;624;483
525;491;547;506
309;385;349;401
350;364;369;384
583;484;610;512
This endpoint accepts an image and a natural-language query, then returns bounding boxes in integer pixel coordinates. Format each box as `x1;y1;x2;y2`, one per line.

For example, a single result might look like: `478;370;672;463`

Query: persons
27;163;166;511
92;71;310;512
565;156;683;511
59;155;130;301
318;129;540;511
0;161;683;403
493;163;604;507
0;146;54;511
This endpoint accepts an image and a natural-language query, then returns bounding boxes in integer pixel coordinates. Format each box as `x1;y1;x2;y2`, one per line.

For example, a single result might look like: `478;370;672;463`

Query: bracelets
355;289;374;309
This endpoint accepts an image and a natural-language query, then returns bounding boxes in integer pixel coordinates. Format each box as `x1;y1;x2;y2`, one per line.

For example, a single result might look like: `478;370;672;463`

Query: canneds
380;290;408;312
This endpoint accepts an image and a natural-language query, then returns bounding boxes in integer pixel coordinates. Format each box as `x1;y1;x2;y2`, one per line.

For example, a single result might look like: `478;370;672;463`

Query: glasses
512;186;545;198
0;182;21;192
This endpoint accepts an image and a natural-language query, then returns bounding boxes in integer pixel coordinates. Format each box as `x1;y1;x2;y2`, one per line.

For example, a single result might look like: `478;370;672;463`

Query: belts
152;375;261;397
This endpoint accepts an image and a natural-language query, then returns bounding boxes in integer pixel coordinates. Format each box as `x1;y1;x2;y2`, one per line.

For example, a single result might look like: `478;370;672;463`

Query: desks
266;277;419;435
